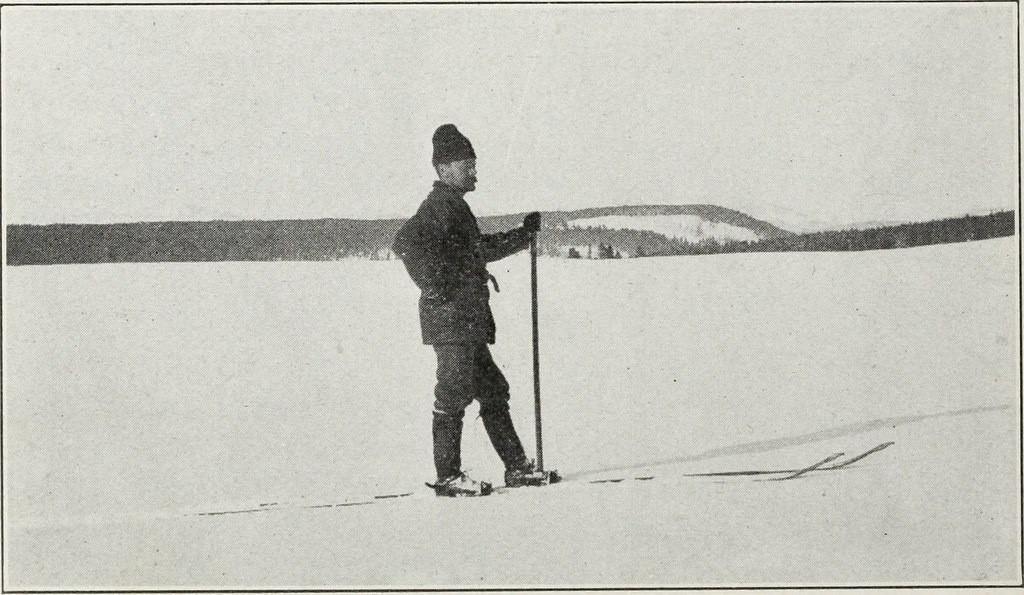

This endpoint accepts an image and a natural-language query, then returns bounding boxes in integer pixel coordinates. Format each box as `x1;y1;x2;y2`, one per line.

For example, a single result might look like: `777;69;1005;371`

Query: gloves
523;212;542;233
431;271;457;302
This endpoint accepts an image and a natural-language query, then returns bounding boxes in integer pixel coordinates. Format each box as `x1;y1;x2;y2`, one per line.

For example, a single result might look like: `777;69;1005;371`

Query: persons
394;124;556;495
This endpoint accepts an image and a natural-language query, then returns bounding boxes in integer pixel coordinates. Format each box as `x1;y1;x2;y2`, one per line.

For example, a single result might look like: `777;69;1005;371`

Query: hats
432;124;476;165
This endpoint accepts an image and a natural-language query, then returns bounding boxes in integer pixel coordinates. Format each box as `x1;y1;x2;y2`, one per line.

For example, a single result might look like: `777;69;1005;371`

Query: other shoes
425;471;492;497
505;458;561;488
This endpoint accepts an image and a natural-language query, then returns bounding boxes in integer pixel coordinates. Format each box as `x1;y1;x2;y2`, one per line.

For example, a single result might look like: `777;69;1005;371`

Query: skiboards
192;432;906;519
